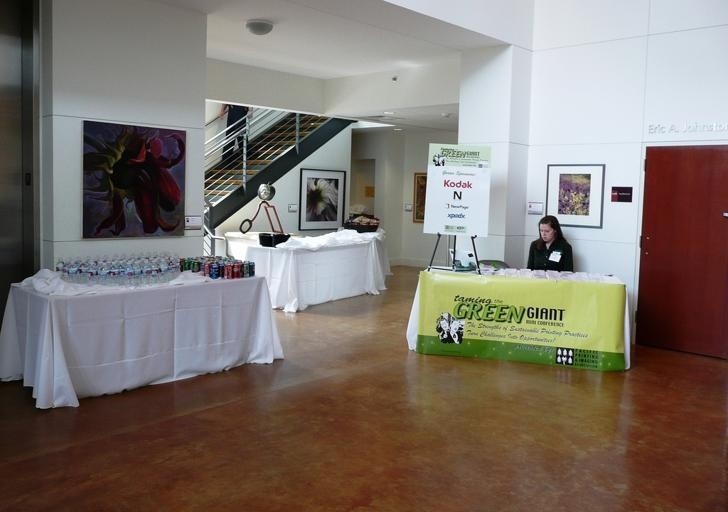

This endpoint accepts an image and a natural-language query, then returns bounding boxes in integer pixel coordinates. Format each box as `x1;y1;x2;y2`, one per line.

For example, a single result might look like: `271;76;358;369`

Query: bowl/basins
259;233;291;247
344;222;378;233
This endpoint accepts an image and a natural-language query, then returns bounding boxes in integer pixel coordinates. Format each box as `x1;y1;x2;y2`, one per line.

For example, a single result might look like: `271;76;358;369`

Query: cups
480;266;605;283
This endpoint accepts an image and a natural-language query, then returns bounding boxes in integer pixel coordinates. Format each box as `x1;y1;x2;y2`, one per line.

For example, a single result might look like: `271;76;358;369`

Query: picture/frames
413;172;426;223
299;168;346;231
546;164;605;228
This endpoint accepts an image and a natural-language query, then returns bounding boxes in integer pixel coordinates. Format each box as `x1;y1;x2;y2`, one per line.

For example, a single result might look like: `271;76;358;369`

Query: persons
218;102;254;171
526;215;574;273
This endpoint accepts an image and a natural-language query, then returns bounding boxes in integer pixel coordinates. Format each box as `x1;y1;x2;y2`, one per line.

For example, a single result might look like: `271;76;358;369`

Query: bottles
54;251;182;289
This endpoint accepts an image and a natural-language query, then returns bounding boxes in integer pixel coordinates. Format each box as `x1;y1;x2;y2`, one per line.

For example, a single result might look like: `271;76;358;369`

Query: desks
0;273;285;409
404;266;630;371
225;228;395;312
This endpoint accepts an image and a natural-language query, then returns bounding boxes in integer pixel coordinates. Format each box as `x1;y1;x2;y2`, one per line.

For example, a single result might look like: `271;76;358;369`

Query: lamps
246;19;273;36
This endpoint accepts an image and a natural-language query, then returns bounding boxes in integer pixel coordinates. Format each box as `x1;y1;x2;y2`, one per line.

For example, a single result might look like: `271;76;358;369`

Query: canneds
179;256;255;279
